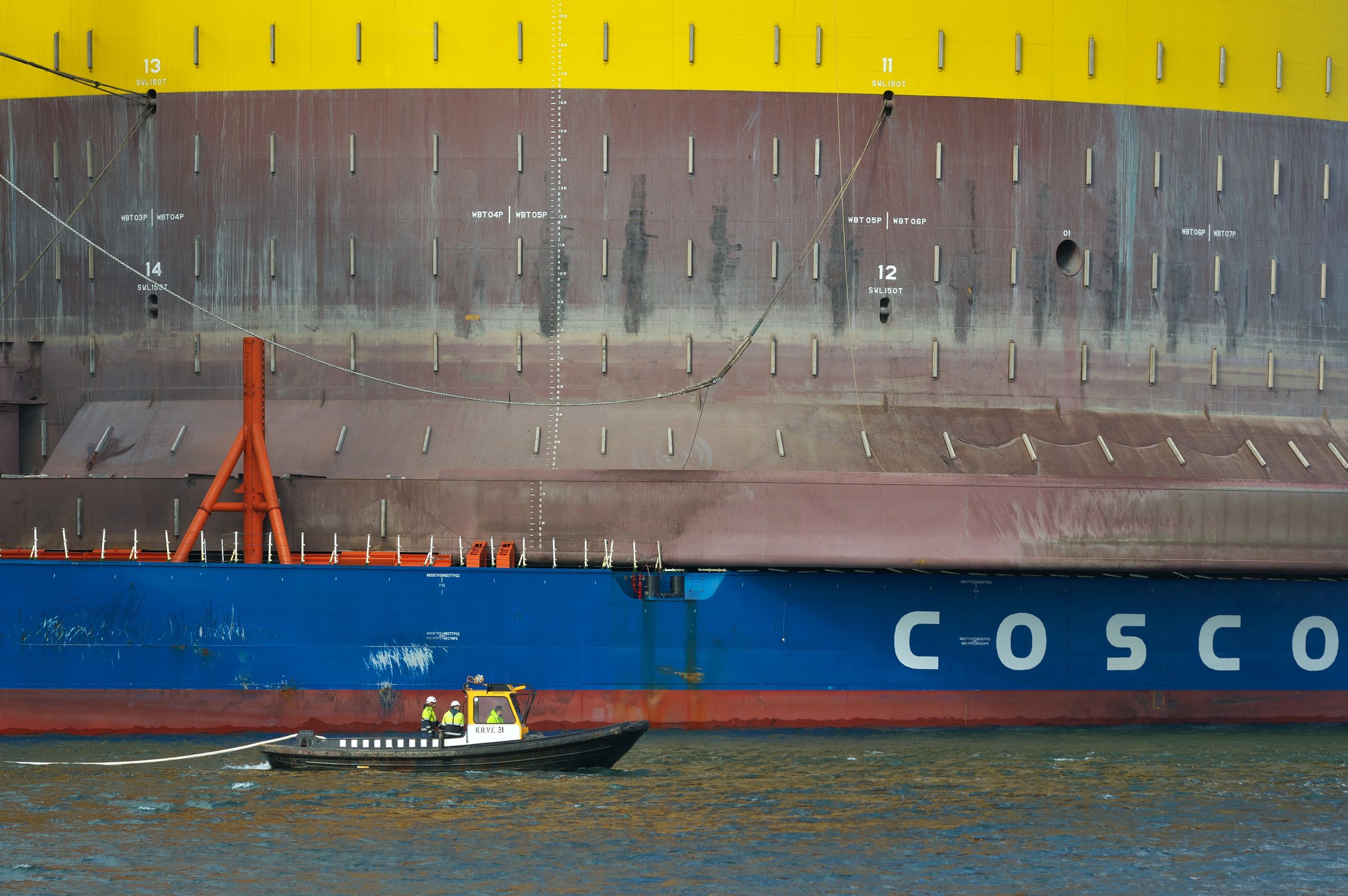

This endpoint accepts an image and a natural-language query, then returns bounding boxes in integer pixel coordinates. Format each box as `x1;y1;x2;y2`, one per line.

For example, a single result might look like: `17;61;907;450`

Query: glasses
497;709;503;712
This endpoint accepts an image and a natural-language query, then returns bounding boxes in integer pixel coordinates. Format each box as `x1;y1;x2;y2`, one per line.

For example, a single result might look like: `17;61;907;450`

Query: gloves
429;733;434;738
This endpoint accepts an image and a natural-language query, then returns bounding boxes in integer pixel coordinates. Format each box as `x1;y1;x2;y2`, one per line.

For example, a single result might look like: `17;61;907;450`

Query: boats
257;674;653;774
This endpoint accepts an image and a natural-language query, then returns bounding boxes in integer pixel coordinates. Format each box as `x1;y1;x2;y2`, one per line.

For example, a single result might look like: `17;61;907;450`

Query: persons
487;705;505;723
441;701;464;739
421;696;442;738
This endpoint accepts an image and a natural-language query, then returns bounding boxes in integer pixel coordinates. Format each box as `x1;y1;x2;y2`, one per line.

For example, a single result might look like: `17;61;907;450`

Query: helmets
426;697;437;704
451;701;461;706
